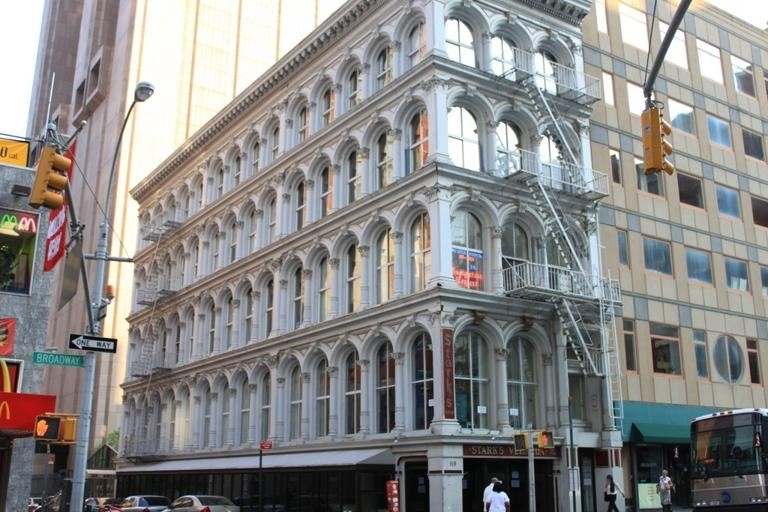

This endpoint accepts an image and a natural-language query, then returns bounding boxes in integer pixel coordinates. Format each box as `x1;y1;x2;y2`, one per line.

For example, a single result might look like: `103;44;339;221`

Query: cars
84;495;243;512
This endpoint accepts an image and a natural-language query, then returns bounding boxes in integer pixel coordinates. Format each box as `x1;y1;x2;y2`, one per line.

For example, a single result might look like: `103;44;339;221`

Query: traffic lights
641;103;674;177
26;138;73;213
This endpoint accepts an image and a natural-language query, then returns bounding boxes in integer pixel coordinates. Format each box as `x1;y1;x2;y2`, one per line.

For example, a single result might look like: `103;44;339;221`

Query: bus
686;407;768;508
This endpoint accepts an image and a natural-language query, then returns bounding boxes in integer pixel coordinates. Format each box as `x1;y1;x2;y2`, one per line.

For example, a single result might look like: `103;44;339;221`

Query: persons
659;469;674;512
482;477;503;512
603;474;625;512
486;482;511;512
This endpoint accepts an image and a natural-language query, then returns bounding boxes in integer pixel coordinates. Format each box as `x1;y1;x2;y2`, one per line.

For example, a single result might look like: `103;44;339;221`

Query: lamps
11;184;32;201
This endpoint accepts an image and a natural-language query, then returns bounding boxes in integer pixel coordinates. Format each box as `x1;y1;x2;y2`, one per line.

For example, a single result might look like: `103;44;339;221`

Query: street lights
65;83;155;510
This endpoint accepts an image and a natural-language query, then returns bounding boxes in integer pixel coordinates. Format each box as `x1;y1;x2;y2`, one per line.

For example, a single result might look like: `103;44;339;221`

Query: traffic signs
68;331;118;354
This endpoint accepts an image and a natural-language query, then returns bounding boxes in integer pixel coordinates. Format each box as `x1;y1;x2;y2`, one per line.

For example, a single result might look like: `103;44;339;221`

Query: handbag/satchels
604;492;611;501
